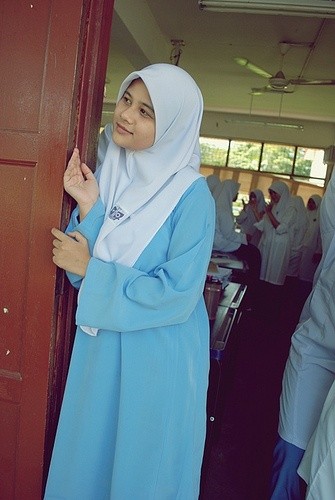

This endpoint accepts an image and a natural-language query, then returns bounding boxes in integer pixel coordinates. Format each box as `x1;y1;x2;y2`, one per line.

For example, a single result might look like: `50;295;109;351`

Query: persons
45;63;215;496
206;167;335;499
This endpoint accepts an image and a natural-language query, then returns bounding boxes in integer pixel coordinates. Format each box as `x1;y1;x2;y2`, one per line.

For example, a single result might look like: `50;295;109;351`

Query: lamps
196;0;335;20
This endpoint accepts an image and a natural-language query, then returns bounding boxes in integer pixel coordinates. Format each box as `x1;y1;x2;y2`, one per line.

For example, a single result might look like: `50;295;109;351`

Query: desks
203;249;250;432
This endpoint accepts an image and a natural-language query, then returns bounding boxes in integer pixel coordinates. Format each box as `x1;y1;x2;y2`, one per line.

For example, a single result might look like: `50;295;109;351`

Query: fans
231;56;335;95
